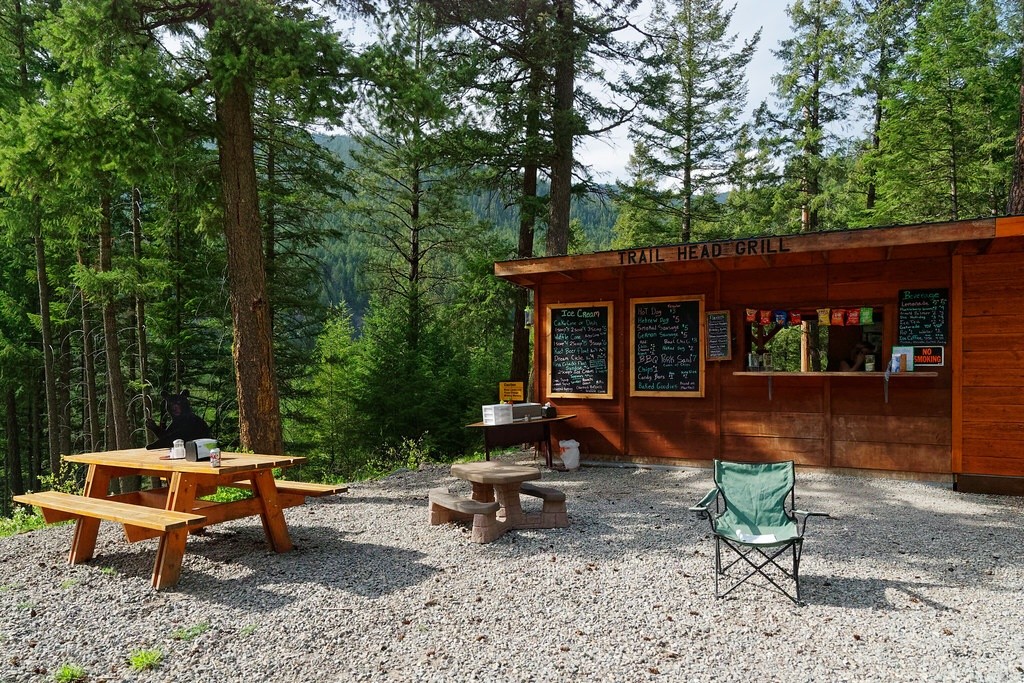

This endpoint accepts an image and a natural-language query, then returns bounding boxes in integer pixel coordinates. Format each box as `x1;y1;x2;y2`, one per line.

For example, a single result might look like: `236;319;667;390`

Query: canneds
890;353;902;372
210;448;221;467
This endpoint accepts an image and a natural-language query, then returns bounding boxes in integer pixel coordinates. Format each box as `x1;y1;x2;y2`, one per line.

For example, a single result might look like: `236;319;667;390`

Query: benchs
220;479;348;498
14;491;207;532
426;488;505;544
521;483;568;529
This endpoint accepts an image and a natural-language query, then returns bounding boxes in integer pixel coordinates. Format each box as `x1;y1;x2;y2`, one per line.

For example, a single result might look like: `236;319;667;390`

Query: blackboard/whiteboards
897;288;949;346
706;311;732;361
629;294;705;398
546;301;614;400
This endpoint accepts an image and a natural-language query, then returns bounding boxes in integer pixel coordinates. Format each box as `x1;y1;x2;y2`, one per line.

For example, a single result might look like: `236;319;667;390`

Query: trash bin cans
559;440;579;468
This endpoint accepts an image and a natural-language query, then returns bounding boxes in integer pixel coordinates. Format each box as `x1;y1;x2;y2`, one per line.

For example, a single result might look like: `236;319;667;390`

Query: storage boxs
513;402;541;422
482;404;513;425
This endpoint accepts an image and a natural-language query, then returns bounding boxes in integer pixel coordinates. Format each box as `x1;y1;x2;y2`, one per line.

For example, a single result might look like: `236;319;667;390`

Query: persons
838;342;880;372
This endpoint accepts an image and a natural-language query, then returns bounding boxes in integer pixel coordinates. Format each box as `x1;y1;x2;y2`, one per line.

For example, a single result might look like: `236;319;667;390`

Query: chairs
690;460;830;603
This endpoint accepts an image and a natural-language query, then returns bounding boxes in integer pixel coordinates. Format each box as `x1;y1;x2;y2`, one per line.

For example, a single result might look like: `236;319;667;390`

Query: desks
467;413;577;468
64;446;308;591
450;461;540;540
